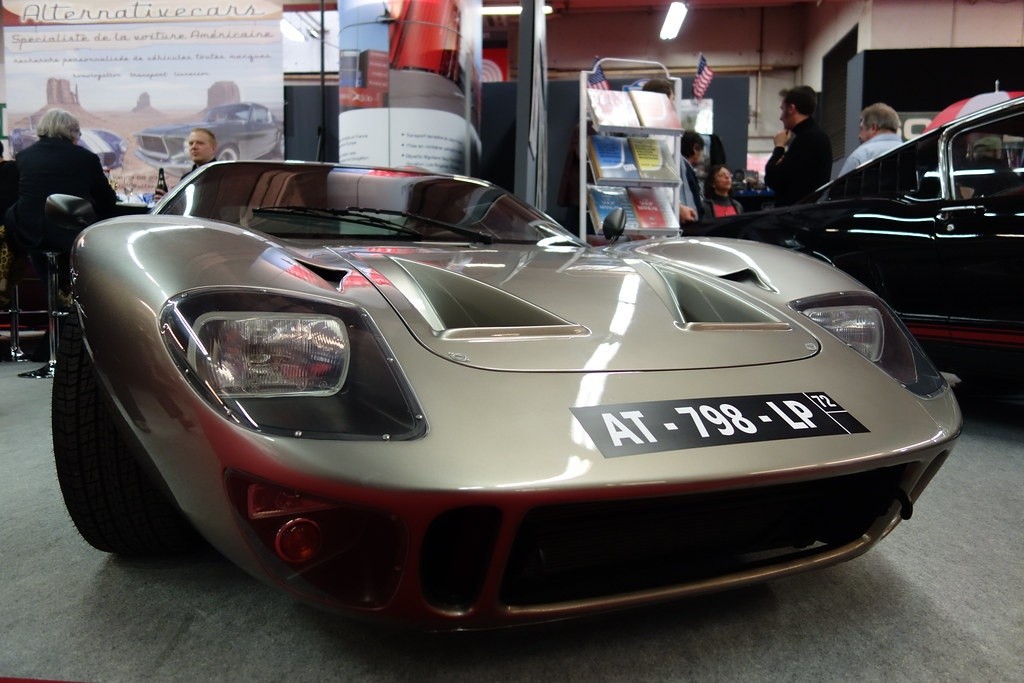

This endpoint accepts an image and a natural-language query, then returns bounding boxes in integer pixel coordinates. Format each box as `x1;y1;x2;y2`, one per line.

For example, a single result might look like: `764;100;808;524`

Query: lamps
658;0;691;40
482;0;554;16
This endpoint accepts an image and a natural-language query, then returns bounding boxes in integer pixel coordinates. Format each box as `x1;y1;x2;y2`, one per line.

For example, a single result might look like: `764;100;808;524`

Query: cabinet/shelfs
578;58;686;244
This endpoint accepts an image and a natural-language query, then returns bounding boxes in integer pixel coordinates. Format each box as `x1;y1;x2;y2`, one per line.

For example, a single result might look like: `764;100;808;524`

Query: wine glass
124;176;132;203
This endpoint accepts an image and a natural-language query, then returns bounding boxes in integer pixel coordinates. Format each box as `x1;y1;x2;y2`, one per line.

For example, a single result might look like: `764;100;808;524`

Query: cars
674;96;1023;403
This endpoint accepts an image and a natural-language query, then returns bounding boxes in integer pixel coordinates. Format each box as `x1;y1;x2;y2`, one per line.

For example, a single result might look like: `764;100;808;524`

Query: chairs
962;153;1021;200
0;193;101;377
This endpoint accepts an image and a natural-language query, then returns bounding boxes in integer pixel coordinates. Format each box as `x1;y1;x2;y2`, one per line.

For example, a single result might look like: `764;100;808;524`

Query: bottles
155;168;168;196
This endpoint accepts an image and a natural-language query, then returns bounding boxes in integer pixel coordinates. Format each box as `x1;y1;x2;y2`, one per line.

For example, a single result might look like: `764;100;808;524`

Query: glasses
714;172;734;180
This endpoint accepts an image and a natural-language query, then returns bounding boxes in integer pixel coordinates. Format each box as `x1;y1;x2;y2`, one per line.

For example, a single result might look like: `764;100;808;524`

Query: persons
0;109;117;362
972;137;1020;197
763;84;832;207
642;79;743;225
154;128;218;204
837;102;905;178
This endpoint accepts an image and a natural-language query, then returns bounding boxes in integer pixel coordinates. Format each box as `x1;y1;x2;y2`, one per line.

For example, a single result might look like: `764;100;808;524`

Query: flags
692;53;714;99
586;58;609;91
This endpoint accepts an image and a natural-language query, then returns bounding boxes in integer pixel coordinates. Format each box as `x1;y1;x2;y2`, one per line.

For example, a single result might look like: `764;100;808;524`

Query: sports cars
34;157;968;638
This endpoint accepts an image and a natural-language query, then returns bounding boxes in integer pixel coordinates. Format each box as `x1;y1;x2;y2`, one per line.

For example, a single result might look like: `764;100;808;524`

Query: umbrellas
922;80;1024;144
